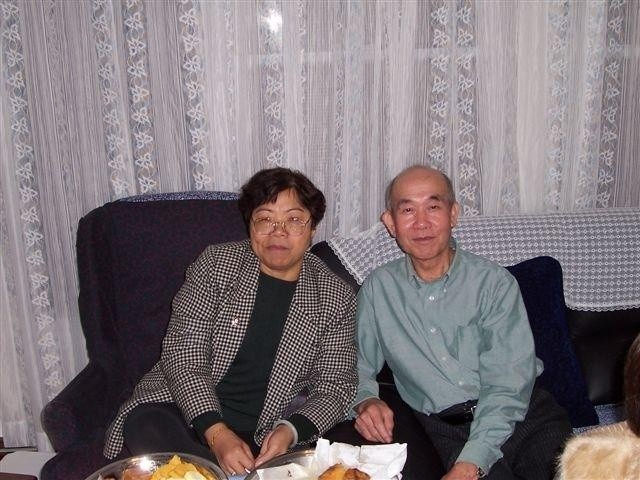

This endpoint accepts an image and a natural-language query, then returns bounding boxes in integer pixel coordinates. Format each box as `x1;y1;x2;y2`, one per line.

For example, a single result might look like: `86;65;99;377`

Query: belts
436;404;478;424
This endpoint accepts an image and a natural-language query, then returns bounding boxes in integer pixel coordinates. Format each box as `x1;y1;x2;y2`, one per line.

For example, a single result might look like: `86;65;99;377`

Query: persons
347;164;571;480
102;167;357;480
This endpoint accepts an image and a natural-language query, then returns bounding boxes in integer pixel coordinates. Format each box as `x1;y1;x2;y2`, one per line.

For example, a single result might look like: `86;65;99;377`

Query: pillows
502;255;599;429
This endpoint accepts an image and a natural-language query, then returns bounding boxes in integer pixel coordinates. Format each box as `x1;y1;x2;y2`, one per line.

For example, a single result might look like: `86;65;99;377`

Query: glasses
252;216;309;236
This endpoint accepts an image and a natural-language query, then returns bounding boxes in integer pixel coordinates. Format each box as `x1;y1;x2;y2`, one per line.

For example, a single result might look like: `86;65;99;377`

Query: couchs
308;207;640;438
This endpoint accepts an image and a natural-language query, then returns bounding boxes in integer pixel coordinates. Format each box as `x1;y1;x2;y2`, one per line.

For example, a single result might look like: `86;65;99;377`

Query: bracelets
210;426;228;450
474;465;485;478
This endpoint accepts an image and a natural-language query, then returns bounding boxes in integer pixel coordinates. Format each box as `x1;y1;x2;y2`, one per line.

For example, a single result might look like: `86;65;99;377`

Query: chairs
40;192;250;480
